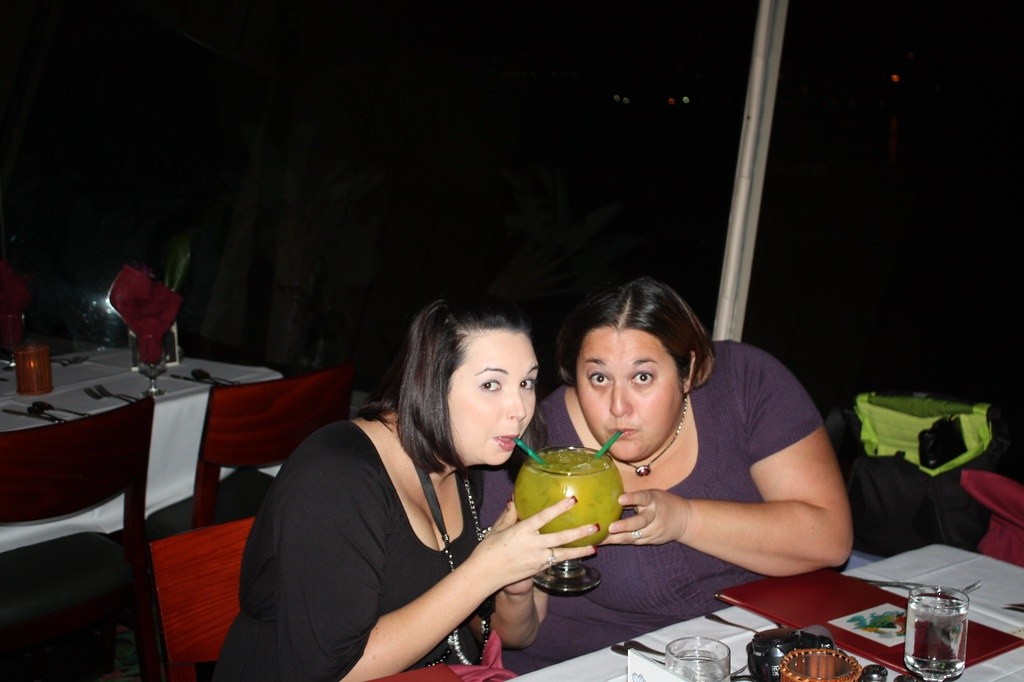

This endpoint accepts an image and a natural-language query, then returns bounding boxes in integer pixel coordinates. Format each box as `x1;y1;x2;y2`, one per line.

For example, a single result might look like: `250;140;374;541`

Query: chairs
0;363;363;682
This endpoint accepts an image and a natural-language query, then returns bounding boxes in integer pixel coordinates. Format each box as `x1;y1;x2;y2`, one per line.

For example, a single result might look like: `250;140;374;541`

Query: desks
0;330;285;557
502;545;1024;682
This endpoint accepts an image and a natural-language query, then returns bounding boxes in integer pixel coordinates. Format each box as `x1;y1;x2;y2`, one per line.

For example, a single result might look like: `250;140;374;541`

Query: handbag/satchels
826;390;1012;555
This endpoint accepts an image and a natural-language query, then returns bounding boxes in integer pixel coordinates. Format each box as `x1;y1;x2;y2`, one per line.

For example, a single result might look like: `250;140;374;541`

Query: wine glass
512;446;624;592
136;334;167;397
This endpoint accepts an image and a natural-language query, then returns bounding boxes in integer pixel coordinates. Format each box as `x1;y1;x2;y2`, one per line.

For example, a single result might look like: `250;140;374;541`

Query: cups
905;586;970;682
664;636;731;682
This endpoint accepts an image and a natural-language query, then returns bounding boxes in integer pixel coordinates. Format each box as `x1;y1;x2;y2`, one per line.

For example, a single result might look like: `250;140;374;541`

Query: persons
206;294;601;682
475;277;854;681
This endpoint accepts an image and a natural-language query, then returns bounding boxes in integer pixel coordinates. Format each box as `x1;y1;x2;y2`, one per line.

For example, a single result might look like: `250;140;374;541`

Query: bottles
862;664;888;682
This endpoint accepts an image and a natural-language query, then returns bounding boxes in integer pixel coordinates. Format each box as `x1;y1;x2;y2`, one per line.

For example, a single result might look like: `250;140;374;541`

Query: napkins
0;257;30;348
110;265;184;364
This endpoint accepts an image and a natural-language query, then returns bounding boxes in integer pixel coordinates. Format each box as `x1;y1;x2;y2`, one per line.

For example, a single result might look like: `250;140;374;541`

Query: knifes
3;408;59;423
170;374;215;385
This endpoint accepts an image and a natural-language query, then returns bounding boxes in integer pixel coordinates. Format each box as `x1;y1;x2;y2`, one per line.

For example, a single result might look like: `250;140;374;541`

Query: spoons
191;369;227;387
32;401;91;417
27;406;66;423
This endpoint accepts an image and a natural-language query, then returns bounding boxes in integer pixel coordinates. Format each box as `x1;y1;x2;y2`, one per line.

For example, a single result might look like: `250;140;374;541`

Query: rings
631;530;642;540
546;549;558;567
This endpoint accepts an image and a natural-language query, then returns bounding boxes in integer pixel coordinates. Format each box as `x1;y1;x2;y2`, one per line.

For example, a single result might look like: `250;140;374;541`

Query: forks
870;579;982;596
626;639;747;678
83;384;139;404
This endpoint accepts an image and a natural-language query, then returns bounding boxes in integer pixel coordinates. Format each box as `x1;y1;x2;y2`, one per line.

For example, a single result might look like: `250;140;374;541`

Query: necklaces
415;456;489;668
612;393;688;478
